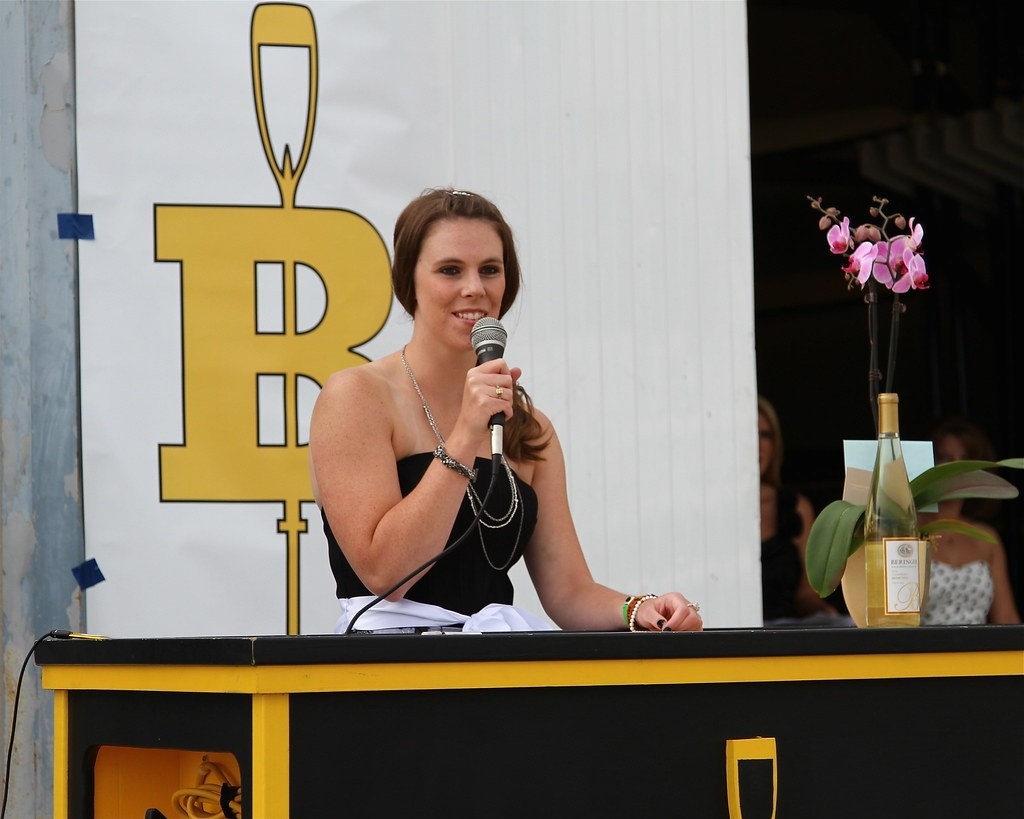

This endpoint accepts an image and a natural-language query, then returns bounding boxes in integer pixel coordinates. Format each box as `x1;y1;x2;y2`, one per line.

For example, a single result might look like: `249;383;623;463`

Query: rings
497;386;503;398
687;602;700;612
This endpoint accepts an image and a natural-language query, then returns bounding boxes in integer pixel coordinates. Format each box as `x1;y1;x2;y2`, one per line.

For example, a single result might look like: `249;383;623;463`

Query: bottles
864;394;920;627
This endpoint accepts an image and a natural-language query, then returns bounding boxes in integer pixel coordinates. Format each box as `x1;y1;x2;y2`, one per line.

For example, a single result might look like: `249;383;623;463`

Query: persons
915;419;1019;624
758;398;838;622
310;191;703;634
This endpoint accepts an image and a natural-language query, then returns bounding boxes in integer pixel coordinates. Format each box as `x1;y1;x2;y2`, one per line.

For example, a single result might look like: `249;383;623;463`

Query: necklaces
401;344;524;570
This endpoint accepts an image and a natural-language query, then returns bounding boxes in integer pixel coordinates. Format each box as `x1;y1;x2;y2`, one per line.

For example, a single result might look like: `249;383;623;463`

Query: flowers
803;191;1024;600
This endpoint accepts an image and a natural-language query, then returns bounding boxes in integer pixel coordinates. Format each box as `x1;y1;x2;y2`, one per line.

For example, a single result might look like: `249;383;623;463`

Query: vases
836;533;931;632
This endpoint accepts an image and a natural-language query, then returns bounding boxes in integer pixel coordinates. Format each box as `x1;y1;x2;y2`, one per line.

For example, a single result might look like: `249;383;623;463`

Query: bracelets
434;446;475;479
623;594;658;633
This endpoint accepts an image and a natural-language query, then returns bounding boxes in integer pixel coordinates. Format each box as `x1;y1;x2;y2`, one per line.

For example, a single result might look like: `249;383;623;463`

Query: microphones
469;316;510;475
